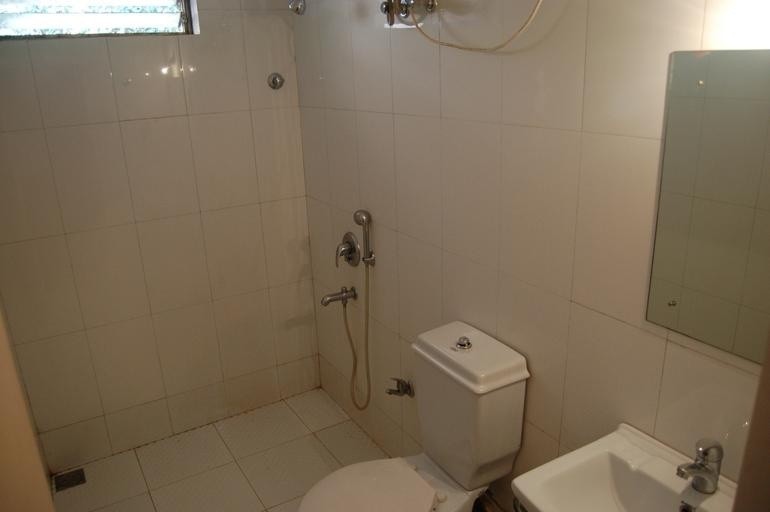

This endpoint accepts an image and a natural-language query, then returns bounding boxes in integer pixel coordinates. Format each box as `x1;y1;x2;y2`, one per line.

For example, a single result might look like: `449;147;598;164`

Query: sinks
512;423;742;511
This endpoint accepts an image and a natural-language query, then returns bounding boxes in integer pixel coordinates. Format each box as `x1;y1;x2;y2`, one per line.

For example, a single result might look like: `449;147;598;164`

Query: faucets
676;437;725;494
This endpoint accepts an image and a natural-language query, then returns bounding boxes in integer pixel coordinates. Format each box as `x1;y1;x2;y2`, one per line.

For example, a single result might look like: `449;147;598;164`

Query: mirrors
643;45;769;368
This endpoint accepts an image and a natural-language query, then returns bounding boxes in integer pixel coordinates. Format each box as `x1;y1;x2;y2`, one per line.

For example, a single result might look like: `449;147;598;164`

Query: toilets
295;318;530;512
